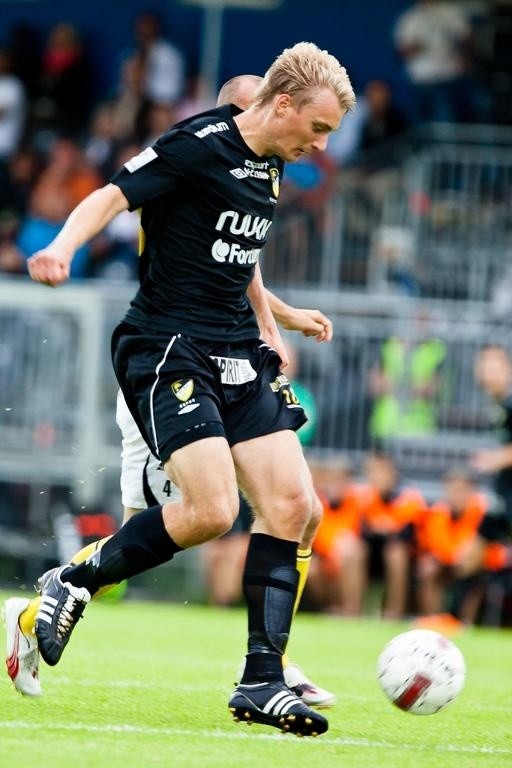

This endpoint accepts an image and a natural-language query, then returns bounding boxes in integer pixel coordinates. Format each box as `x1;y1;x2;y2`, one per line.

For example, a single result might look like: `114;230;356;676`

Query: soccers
376;628;465;717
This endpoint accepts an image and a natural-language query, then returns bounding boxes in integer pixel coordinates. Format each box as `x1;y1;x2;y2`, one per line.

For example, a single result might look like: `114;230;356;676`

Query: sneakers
3;597;43;697
237;656;337;707
228;680;330;737
34;564;92;666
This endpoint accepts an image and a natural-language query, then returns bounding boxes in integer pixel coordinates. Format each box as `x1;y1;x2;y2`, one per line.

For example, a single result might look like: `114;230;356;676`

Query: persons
23;35;360;737
2;68;342;714
2;1;512;626
